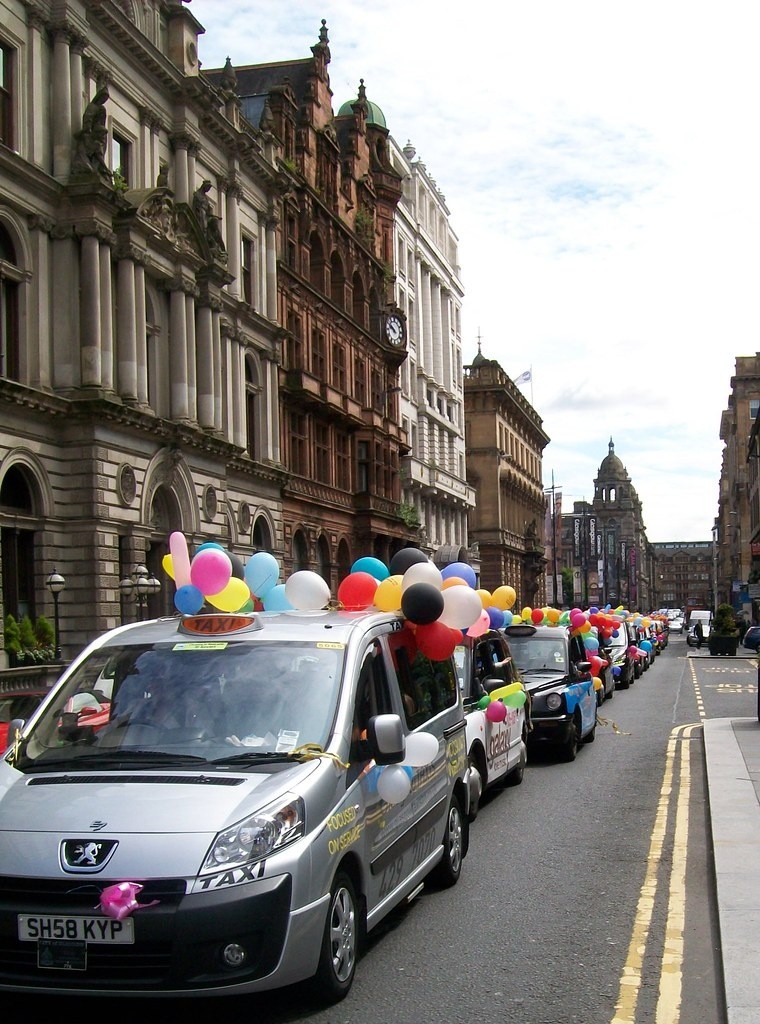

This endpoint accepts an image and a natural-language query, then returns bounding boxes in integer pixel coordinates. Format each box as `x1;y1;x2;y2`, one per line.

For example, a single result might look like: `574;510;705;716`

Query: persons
736;617;758;645
694;619;703;649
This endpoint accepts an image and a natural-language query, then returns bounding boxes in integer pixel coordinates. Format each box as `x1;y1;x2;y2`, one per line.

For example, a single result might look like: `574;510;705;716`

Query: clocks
386;317;403;345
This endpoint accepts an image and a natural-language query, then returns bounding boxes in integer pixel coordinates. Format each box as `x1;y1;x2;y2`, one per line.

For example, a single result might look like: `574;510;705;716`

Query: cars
453;629;533;823
627;620;669;679
605;621;634;689
742;627;760;653
94;652;151;700
0;687;113;756
1;609;472;1004
492;624;598;761
659;609;686;634
576;627;615;707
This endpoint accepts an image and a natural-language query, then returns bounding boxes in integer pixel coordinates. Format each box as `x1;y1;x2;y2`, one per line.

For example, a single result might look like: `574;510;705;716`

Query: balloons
479;683;527;722
513;604;669;689
378;731;440;804
162;531;515;661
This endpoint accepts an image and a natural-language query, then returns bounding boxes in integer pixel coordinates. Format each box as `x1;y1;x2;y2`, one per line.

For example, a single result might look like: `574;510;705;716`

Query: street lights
708;588;712;625
44;568;65;660
118;563;161;621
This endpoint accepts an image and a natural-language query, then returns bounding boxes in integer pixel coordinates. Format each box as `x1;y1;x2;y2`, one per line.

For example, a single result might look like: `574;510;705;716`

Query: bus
683;597;707;613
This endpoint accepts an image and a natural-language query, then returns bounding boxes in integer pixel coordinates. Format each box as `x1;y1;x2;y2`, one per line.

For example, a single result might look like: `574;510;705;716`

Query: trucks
684;611;713;645
